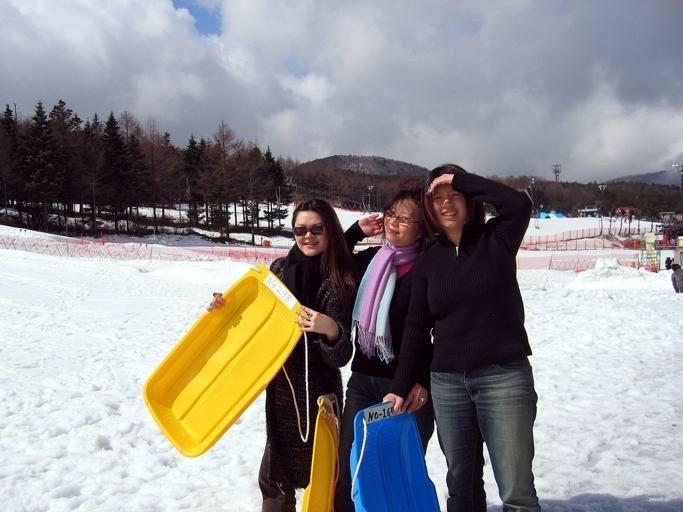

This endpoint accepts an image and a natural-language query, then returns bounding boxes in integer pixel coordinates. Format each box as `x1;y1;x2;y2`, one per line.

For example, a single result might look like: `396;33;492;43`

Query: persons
420;165;539;511
671;263;683;293
332;188;437;511
206;201;354;512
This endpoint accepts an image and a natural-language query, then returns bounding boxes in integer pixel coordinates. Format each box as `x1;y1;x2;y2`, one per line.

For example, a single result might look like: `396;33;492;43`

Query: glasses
292;227;326;236
385;209;421;224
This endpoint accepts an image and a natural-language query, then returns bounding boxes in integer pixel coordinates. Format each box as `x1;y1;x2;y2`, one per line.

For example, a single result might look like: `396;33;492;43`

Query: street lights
596;183;609;217
365;185;375;214
528;177;536;217
670;161;682;213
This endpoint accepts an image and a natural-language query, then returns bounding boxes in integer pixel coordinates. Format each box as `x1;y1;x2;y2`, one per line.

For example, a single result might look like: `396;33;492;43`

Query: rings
420;398;424;402
307;314;312;320
212;293;217;296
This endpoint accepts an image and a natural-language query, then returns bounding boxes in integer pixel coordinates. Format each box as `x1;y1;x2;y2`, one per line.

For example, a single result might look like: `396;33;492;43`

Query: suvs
655;223;663;234
